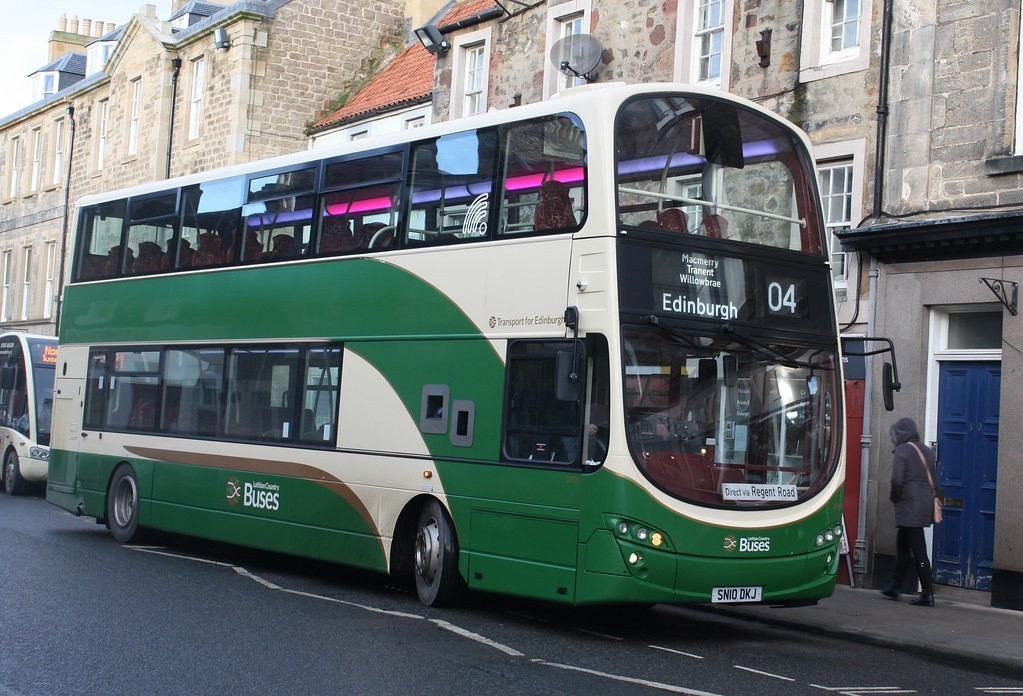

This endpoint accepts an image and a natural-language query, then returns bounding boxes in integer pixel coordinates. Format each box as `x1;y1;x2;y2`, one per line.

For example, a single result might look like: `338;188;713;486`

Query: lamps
213;25;232;52
416;14;455;57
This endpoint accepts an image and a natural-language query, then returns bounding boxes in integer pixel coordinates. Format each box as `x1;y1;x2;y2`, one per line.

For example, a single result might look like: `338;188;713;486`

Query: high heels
909;588;934;606
882;586;900;598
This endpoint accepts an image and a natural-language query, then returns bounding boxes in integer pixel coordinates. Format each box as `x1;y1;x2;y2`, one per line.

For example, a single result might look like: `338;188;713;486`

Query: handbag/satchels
931;497;943;524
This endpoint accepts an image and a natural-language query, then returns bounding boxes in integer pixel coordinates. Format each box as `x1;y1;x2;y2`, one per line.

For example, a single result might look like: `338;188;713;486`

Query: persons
878;417;943;608
515;374;599;463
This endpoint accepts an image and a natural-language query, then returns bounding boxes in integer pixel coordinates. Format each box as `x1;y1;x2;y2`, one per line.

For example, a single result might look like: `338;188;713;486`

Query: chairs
91;383;333;443
99;158;731;276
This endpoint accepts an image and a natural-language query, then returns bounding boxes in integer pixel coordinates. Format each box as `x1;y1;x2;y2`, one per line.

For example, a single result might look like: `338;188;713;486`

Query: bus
45;81;902;609
0;332;59;496
